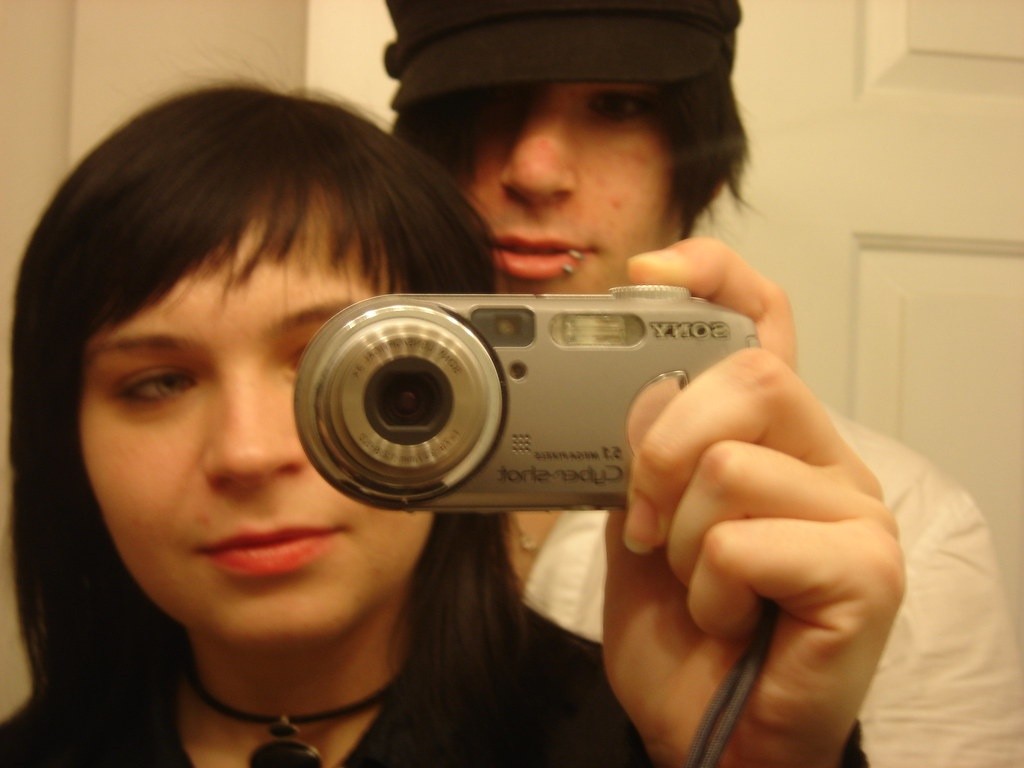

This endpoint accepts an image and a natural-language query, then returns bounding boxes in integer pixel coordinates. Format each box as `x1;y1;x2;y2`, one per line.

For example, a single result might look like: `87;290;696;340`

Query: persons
0;0;1024;768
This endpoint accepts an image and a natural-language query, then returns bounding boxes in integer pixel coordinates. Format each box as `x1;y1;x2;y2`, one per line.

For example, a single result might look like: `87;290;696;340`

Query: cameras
294;284;759;511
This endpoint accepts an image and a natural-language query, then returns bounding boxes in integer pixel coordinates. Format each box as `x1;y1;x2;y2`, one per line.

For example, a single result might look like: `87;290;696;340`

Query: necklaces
193;675;393;768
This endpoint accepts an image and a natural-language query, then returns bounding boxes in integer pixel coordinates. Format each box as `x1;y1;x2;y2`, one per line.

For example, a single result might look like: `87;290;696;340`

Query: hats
382;0;740;114
